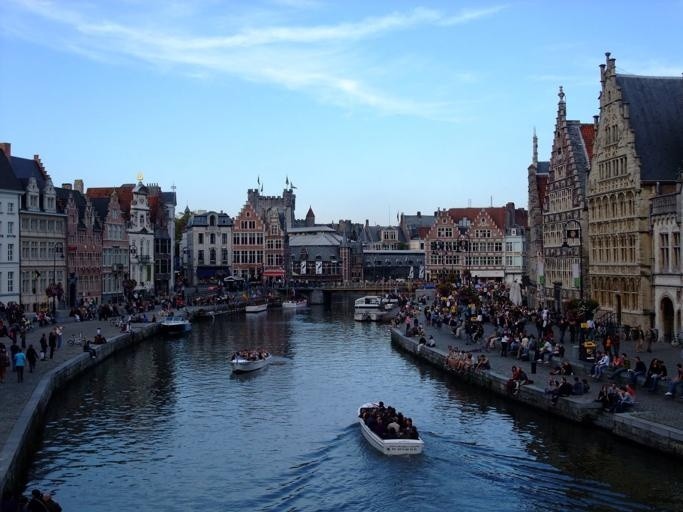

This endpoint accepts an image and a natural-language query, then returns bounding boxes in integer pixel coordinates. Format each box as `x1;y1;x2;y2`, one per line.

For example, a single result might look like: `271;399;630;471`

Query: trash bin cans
585;341;597;360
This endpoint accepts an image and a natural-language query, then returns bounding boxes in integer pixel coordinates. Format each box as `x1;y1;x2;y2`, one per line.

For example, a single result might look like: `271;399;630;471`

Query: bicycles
67;334;83;347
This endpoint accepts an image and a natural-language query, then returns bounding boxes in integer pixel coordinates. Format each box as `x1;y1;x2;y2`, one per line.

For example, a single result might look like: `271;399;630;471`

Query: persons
179;278;308;305
501;318;681;415
0;490;61;512
2;304;106;382
230;347;269;361
70;294;190;333
362;275;567;371
362;400;419;439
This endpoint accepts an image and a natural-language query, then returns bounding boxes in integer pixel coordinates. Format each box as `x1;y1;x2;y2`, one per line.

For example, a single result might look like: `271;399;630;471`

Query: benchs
543;355;669;388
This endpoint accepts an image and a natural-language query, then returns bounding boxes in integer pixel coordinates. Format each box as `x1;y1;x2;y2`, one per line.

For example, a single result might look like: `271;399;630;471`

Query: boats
160;316;191;332
230;352;272;374
246;304;268;313
282;300;306;308
354;296;400;321
357;403;424;456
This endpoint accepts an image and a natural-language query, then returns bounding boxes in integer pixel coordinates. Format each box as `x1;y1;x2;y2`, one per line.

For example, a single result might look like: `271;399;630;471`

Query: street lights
454;234;471;291
52;241;64;315
560;219;583;302
182;246;191;301
128;244;137;280
